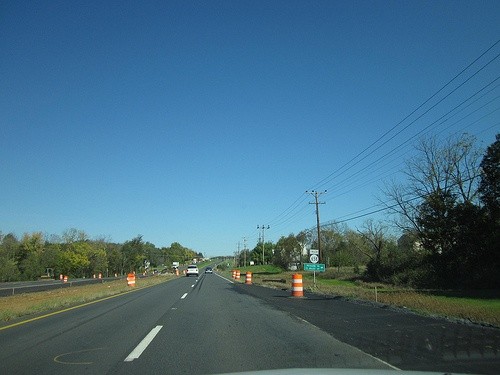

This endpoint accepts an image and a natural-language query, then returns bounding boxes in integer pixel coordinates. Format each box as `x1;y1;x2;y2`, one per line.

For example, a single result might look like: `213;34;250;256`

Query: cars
205;266;213;274
154;268;158;273
162;265;178;273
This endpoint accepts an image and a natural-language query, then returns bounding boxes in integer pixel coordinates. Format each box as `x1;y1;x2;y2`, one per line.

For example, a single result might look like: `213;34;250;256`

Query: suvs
186;265;200;277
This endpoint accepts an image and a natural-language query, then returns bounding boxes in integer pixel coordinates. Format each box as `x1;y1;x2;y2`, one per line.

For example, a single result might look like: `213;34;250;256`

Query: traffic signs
303;263;326;273
